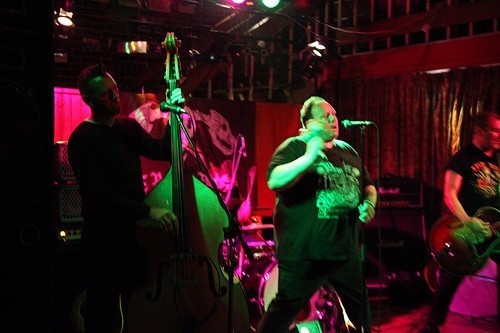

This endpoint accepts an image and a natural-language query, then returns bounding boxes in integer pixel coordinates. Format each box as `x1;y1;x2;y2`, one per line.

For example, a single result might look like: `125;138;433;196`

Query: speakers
37;142;76;182
359;208;427;294
47;184;84;224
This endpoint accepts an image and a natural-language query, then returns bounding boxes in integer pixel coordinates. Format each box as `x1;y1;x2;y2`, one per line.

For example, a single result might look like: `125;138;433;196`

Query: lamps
296;40;328;82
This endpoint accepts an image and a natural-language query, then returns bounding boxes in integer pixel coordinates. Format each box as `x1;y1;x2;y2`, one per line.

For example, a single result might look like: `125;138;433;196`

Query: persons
417;112;500;332
250;96;378;333
67;64;185;333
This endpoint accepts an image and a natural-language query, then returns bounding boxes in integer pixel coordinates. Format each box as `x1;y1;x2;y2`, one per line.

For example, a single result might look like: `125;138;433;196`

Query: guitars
428;206;499;276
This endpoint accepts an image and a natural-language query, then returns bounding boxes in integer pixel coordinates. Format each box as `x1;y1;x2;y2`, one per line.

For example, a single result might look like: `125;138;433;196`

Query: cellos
128;30;251;332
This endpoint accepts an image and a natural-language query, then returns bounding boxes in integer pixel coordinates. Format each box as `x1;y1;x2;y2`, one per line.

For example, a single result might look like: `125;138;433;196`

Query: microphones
340;120;375;129
160;102;188;114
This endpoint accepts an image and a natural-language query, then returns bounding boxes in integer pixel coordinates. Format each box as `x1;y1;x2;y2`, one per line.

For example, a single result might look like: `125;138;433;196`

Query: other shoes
419;325;439;333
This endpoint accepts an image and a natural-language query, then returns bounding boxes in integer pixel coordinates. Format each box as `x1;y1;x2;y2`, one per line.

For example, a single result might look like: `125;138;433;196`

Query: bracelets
363;199;377;210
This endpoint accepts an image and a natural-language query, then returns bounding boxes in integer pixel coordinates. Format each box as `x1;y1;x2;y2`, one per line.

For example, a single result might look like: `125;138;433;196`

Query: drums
239;250;336;330
